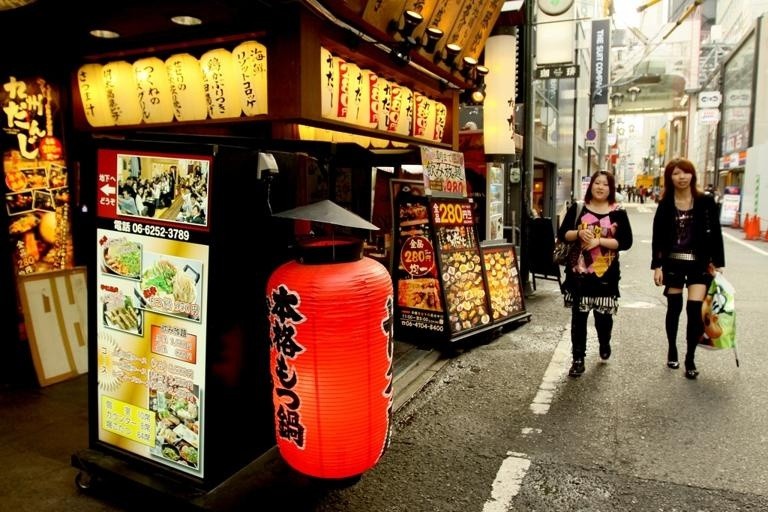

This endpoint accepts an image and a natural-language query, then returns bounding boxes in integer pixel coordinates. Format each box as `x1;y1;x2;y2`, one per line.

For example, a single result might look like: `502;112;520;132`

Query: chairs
527;216;563;292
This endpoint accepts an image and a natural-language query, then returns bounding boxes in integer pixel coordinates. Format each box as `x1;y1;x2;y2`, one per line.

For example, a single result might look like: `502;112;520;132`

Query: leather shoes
686;367;699;379
569;358;585;375
598;342;611;360
667;361;680;369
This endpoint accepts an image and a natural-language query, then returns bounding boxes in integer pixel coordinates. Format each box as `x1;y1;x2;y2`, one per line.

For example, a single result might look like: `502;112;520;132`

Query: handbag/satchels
551;200;584;266
698;272;736;350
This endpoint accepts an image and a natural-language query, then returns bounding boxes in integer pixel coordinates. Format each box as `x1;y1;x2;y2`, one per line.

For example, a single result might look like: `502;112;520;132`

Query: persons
615;182;665;204
704;183;721;200
557;170;633;376
651;157;725;378
117;165;208;225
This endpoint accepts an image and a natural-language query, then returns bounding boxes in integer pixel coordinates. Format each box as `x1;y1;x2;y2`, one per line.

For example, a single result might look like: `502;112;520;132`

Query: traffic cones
731;211;740;228
741;212;768;242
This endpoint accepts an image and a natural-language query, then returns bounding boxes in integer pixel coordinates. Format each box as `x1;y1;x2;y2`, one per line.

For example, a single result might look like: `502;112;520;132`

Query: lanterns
78;64;115;127
101;61;143;125
231;41;268;117
378;77;413;136
165;53;207;121
413;91;447;142
321;46;378;128
132;57;174;123
199;48;242;119
264;234;396;489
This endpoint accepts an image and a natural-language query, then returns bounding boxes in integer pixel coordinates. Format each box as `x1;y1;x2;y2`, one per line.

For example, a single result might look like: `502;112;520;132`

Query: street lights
587;74;644;177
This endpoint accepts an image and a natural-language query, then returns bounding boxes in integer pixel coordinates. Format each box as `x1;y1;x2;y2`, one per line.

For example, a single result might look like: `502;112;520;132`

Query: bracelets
599;237;600;246
577;229;580;239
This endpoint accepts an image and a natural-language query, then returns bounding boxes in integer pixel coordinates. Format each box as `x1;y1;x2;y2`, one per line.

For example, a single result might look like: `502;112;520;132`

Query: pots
102;240;140;278
103;297;142;335
133;264;202;319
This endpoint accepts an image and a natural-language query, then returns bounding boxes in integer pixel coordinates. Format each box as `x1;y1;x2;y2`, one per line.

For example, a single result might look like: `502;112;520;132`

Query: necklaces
675;197;693;245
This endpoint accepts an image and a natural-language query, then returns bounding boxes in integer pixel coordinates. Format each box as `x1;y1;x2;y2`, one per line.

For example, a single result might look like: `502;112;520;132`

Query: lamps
387;10;488;103
